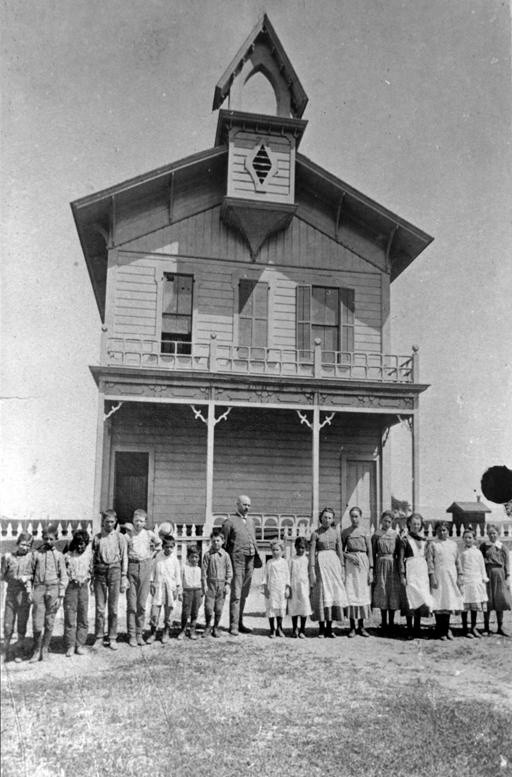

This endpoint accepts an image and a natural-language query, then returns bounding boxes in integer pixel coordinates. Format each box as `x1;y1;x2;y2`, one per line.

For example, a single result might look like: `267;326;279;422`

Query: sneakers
318;628;337;638
109;639;118;651
14;649;24;662
231;625;252;635
147;634;156;644
294;629;299;637
177;631;186;640
93;638;105;650
441;624;508;641
162;634;169;644
42;632;50;660
129;634;146;647
300;633;306;638
382;621;416;630
276;627;285;637
190;633;198;640
360;628;369;637
1;654;8;663
30;631;42;663
66;646;75;657
348;629;356;637
75;646;87;654
268;629;277;638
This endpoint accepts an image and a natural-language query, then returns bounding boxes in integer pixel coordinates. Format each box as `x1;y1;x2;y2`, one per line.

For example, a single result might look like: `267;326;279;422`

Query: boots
201;615;212;638
213;613;221;638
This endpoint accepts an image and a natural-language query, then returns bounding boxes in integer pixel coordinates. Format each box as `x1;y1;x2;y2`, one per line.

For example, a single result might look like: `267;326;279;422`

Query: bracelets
121;571;126;576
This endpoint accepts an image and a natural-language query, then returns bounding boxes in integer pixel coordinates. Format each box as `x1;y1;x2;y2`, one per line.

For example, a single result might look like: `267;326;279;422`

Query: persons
223;493;263;636
0;530;33;662
26;526;69;664
89;508;128;650
60;530;91;658
